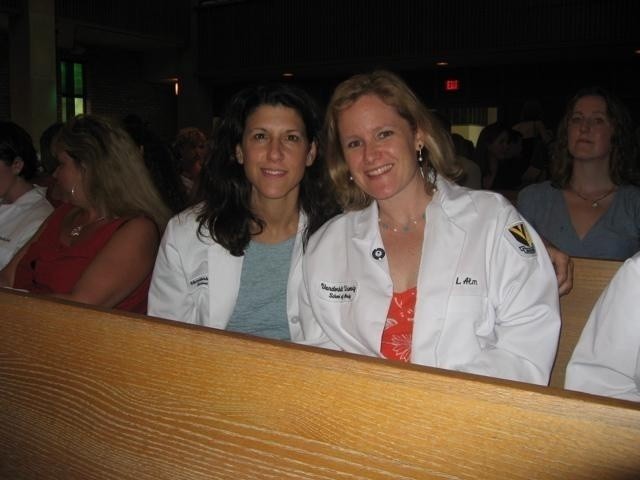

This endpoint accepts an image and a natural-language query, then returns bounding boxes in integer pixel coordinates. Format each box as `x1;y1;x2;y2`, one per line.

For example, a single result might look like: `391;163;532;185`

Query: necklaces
69;215;106;238
380;214;426;233
571;187;617;210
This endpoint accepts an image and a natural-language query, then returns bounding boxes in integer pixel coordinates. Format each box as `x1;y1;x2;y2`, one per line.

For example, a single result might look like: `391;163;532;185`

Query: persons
1;117;56;271
431;113;566;197
41;122;65;187
120;115;211;197
296;68;563;386
145;85;341;345
563;251;640;402
0;112;171;314
515;88;640;261
